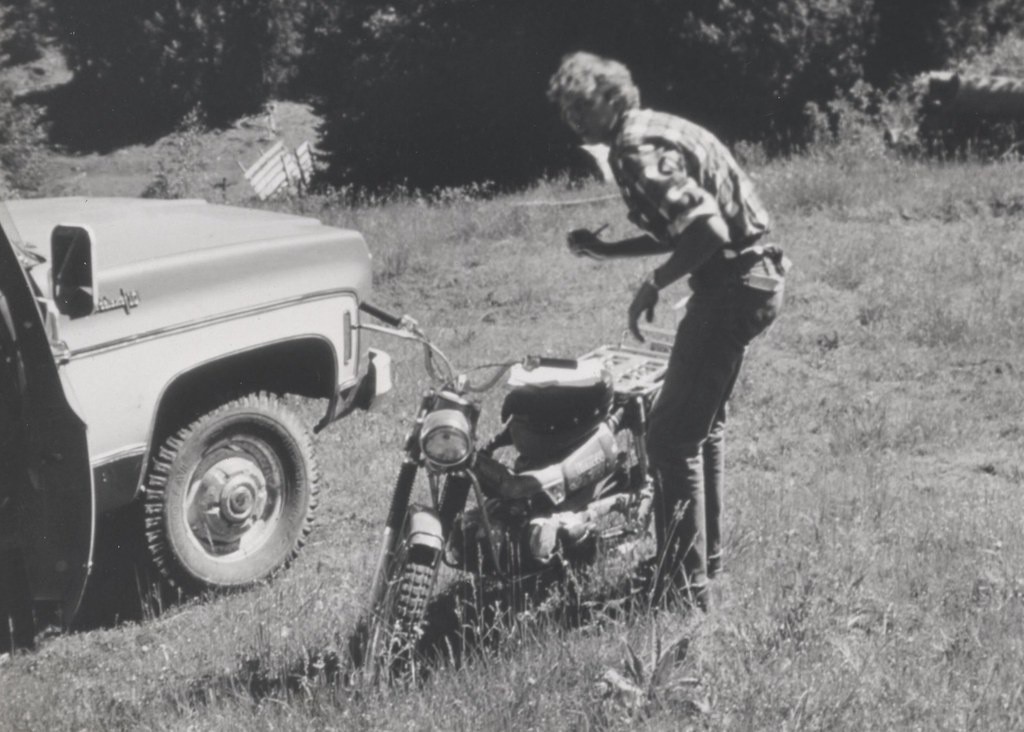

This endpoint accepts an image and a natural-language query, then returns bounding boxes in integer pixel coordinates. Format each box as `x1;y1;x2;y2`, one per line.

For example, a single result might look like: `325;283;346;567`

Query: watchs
645;269;660;291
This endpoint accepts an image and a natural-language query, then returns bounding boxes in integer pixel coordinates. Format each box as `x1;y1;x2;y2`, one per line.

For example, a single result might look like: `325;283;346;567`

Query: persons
549;52;785;614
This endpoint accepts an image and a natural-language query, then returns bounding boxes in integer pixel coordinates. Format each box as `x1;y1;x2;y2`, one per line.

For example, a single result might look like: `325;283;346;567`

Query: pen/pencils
591;221;611;237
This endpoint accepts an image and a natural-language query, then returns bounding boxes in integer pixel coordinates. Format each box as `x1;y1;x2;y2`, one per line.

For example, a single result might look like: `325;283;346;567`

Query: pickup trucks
0;197;392;638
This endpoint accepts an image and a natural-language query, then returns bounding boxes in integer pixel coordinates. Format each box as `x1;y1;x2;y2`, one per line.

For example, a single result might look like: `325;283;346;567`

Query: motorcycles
350;300;678;684
